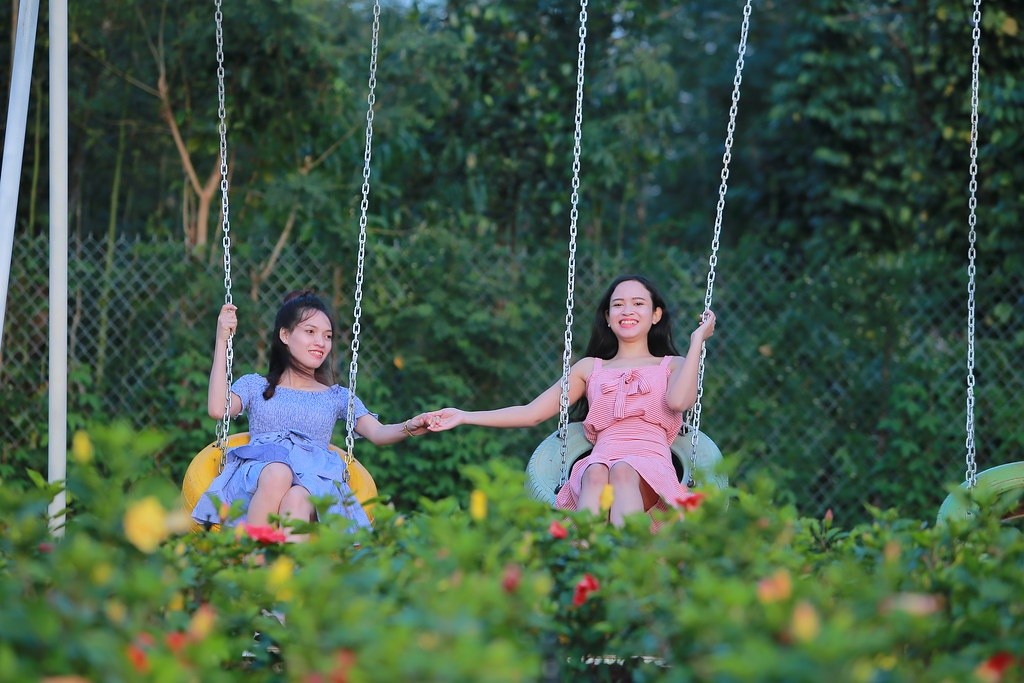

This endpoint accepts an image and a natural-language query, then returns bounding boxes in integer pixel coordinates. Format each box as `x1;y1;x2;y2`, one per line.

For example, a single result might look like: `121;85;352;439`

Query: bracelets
401;419;416;437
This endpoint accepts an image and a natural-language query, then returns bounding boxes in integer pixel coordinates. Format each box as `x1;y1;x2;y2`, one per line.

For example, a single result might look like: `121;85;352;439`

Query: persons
190;294;452;663
427;275;716;548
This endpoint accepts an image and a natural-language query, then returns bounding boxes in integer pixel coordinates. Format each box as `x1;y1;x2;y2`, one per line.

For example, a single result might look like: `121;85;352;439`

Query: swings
520;0;758;528
179;0;387;555
